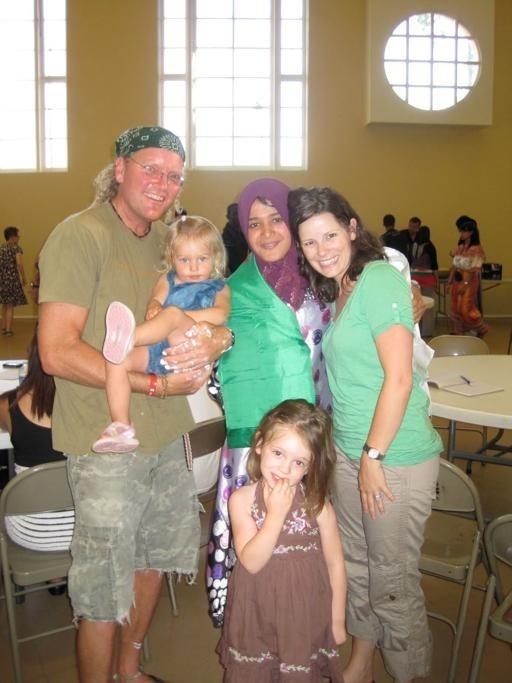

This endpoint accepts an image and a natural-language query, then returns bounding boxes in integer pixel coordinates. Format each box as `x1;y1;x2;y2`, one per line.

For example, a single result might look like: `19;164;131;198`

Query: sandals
92;421;139;453
1;327;13;337
103;300;135;364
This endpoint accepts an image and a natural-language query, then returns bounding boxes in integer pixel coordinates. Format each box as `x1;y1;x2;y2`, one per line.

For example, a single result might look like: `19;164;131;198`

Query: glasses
126;155;184;187
13;235;21;238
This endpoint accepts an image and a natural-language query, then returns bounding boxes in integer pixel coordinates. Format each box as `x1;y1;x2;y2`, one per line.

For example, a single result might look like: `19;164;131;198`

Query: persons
205;176;428;630
1;227;27;338
37;123;236;683
398;215;439;339
215;398;348;682
445;216;491;339
0;314;76;596
90;214;232;453
287;184;444;682
379;213;412;266
221;205;252;275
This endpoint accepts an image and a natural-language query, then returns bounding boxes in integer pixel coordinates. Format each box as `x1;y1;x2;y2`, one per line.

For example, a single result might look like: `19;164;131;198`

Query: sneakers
477;325;492;339
45;580;67;596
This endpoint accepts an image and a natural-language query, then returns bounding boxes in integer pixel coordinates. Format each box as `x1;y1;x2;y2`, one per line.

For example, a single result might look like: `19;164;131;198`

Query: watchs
361;443;385;461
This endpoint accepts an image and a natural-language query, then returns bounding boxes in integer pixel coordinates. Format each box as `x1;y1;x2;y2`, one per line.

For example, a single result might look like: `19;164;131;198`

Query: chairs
0;261;512;683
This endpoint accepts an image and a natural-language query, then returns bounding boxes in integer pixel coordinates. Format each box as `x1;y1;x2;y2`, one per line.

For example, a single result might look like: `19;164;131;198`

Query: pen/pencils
461;376;470;385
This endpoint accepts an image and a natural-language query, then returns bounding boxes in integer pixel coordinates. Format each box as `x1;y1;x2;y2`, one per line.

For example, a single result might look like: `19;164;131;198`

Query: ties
415;244;419;259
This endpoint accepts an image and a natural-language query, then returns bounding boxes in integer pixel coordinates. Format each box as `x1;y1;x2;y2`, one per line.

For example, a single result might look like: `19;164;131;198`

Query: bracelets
161;374;168;399
149;370;157;398
